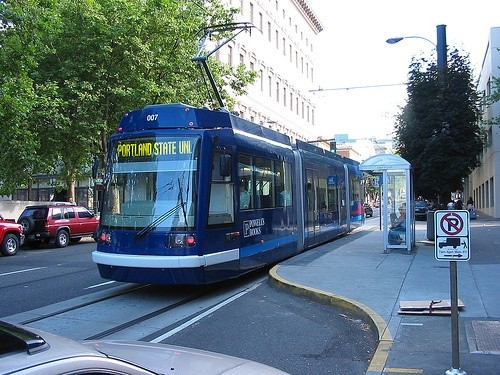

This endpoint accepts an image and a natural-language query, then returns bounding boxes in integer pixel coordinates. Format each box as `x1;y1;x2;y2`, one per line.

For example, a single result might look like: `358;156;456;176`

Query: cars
364;203;373;218
0;319;294;374
415;199;427;220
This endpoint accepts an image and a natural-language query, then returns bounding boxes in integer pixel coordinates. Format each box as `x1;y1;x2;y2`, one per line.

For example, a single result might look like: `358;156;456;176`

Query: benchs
390;231;405;245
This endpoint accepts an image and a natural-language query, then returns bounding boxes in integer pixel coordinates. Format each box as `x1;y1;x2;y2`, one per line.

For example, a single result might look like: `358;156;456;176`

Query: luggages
470;209;476;220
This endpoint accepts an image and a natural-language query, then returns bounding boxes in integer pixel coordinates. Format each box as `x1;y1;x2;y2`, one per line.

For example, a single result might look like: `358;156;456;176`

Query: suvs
17;204;100;247
0;215;23;257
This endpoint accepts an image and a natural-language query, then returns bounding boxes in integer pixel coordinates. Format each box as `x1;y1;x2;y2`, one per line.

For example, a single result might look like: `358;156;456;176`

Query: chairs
249;193;284;209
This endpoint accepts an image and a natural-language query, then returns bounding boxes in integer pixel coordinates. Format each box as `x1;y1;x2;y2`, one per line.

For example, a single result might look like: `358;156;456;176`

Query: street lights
387;36;451;211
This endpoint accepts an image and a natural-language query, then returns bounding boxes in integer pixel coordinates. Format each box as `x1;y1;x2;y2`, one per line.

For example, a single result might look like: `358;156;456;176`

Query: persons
452;196;474;210
388;203;406;250
69;198;76;205
239;188;250;209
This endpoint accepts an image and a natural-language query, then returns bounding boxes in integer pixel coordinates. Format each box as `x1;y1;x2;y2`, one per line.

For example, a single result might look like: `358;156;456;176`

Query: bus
92;103;365;287
